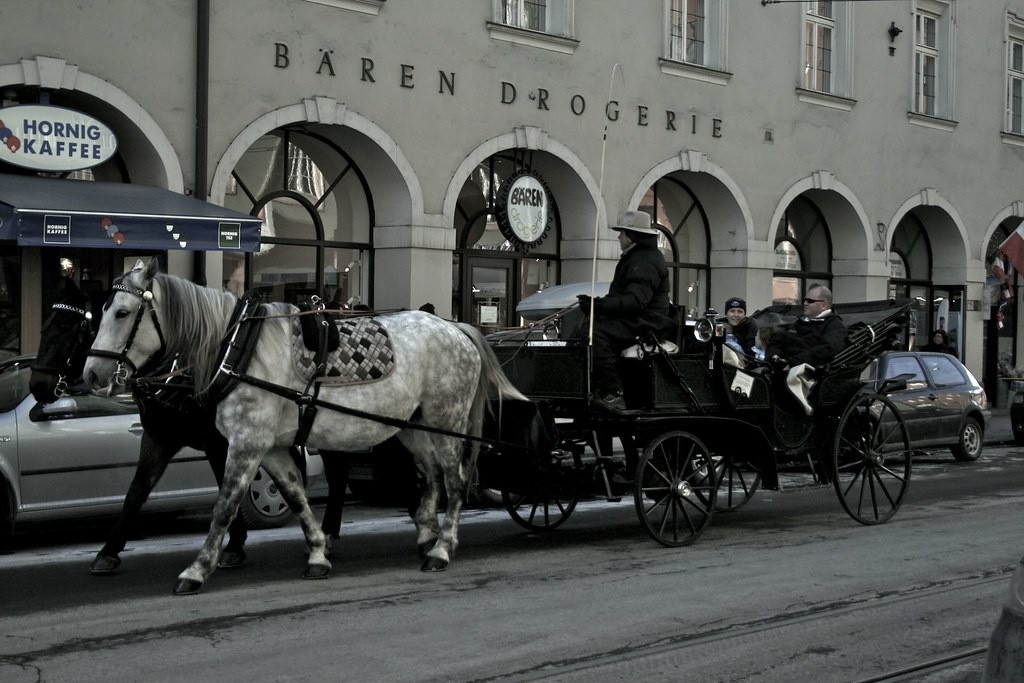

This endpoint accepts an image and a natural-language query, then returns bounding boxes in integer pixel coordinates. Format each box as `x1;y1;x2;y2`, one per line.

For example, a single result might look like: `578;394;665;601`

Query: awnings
0;173;263;254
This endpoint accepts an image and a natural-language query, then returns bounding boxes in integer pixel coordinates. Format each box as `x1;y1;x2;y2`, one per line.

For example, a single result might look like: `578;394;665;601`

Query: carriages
33;253;926;596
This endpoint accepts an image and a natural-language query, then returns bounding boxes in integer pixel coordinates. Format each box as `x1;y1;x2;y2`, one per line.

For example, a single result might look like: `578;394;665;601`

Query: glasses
803;297;825;304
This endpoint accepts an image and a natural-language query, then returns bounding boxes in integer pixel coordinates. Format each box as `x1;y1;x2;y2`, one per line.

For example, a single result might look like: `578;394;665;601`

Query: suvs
479;277;743;372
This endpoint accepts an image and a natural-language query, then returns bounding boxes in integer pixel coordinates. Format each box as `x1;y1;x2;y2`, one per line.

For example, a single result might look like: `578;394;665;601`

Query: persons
569;212;669;410
716;283;847;375
919;330;958;359
419;302;439;316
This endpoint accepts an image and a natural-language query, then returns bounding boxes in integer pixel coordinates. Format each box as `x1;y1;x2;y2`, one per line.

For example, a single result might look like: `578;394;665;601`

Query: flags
988;220;1024;297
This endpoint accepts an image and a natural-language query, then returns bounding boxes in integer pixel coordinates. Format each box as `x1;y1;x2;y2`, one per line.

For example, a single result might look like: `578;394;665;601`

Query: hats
611;210;659;236
725;297;747;315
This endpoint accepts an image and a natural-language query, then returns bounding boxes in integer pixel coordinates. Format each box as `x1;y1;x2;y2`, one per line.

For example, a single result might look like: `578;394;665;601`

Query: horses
28;257;529;596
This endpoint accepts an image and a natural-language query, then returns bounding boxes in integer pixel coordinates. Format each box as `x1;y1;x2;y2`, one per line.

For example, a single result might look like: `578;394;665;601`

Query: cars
0;351;330;554
858;350;994;464
